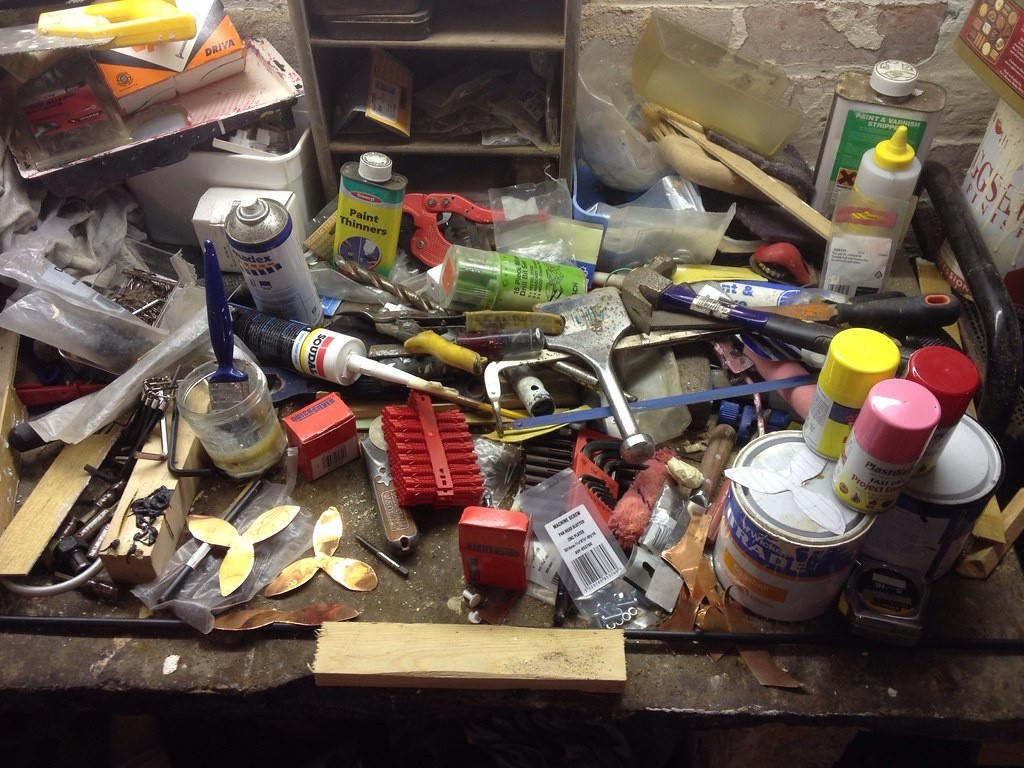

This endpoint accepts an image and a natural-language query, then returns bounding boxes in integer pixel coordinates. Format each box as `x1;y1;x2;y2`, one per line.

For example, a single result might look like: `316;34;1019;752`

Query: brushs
201;240;260;448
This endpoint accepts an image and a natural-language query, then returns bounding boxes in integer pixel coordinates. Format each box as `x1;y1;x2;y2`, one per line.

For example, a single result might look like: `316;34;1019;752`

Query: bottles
331;151;407;281
831;377;942;514
903;347;979;477
846;126;921;236
224;196;323;329
807;59;947;229
438;244;588;312
802;328;900;462
227;302;459;399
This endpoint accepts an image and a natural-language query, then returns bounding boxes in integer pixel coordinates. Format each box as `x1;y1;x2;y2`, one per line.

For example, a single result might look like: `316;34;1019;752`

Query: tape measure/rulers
839;557;934;646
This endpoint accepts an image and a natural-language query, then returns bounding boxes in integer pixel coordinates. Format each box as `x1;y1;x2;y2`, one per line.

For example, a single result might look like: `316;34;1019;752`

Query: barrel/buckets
713;431;879;622
934;98;1024;313
860;413;1005;583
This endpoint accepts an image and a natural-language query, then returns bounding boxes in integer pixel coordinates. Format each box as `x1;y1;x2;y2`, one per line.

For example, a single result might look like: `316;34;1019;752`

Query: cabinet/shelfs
287;0;583;211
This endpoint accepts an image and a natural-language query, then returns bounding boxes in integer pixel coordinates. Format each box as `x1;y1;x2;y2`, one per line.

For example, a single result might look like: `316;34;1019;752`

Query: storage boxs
281;392;359;481
88;0;247;115
191;186;305;274
632;12;804;160
125;96;324;248
952;0;1024;118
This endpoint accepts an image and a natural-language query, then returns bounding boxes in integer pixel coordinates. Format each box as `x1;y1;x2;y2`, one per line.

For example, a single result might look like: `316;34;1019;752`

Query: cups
175;358;287;478
